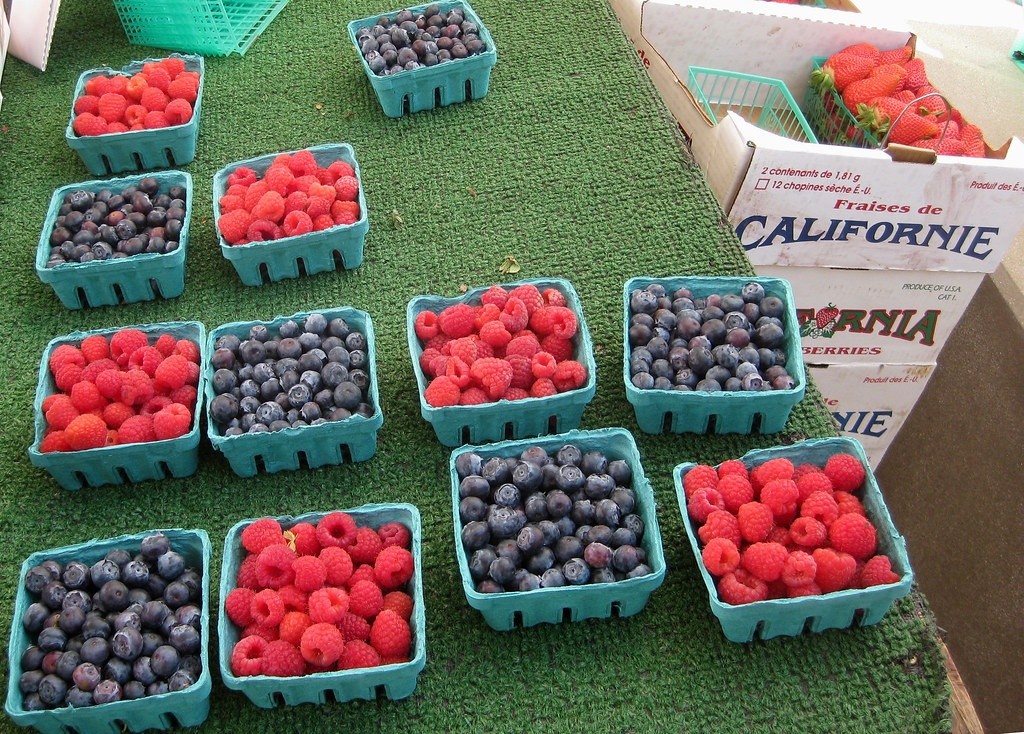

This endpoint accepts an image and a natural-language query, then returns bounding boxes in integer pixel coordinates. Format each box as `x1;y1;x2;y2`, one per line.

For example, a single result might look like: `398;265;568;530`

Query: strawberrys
808;42;987;158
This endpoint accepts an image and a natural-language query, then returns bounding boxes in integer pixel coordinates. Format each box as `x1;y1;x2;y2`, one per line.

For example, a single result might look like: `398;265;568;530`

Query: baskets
4;530;211;734
35;170;193;309
206;306;385;478
216;503;427;708
448;427;666;633
673;436;913;643
688;66;817;146
348;0;497;117
212;142;368;287
29;321;206;489
116;0;290;58
66;52;205;176
621;276;806;435
404;277;597;445
805;58;887;155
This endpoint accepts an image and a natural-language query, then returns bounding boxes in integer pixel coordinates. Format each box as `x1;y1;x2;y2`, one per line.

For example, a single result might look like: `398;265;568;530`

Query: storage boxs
620;0;1023;481
112;0;289;58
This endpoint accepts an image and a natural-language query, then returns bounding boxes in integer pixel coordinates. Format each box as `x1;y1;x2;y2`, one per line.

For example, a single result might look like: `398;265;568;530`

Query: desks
2;1;984;734
